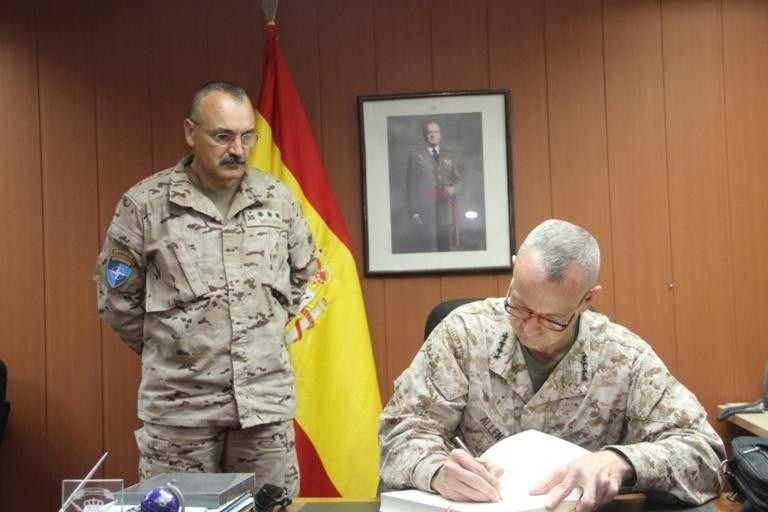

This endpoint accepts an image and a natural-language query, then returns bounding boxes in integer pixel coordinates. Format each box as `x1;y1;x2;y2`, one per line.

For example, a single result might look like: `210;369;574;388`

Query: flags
244;22;385;501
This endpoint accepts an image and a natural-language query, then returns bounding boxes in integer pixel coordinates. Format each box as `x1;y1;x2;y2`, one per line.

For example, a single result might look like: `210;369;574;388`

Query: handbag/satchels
721;435;768;512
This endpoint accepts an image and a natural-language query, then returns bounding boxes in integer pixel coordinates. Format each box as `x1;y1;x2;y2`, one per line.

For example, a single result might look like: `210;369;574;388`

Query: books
377;428;596;512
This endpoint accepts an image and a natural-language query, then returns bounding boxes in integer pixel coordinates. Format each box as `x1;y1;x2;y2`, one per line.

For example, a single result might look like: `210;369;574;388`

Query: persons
405;121;466;253
91;79;319;499
375;216;727;511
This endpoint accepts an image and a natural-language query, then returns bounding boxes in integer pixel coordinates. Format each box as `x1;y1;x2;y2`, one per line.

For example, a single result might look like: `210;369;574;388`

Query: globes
141;478;185;512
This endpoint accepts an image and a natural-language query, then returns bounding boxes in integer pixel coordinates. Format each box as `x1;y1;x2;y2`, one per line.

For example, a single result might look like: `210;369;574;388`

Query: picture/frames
356;87;517;279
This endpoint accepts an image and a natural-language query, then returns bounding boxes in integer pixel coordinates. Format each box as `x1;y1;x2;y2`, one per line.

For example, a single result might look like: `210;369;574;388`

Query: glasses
189;120;260;148
502;278;589;332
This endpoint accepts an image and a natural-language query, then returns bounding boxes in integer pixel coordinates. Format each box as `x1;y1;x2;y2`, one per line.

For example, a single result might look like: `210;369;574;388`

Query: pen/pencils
452;436;503;501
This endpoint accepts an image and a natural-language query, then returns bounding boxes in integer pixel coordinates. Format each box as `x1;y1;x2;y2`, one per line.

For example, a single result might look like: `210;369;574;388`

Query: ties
431;149;439;161
